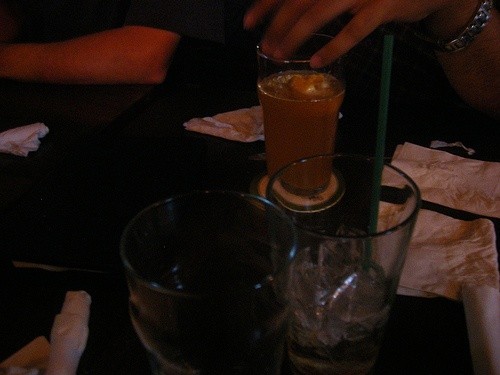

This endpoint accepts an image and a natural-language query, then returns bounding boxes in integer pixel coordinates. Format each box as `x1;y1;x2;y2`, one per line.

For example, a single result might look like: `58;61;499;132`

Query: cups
266;156;419;375
120;190;296;375
255;37;346;193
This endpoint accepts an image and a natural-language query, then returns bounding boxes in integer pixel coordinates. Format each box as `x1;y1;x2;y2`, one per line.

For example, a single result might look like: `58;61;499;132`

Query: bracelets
413;0;493;54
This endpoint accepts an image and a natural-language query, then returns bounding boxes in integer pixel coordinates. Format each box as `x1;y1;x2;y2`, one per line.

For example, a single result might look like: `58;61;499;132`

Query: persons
0;0;225;211
243;0;500;119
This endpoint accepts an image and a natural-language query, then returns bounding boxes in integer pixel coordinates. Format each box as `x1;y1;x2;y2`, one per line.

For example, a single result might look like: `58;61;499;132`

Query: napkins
370;142;500;375
0;122;48;156
184;107;263;143
44;291;92;375
